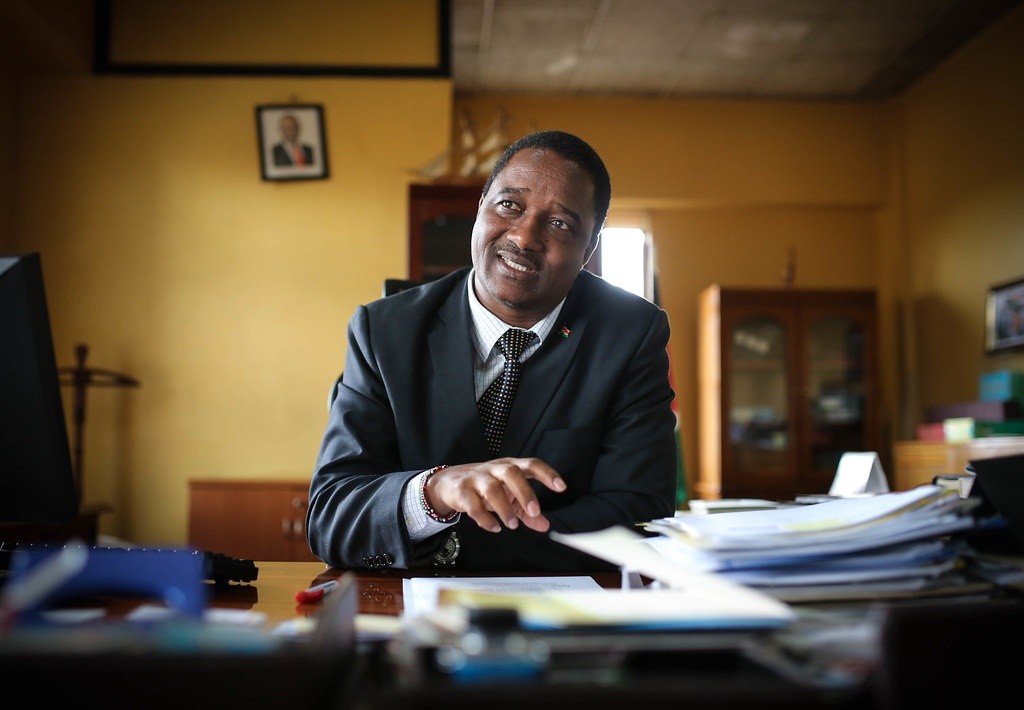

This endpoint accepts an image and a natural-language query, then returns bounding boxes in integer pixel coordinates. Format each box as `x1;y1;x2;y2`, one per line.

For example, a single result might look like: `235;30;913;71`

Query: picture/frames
984;276;1024;359
255;103;328;182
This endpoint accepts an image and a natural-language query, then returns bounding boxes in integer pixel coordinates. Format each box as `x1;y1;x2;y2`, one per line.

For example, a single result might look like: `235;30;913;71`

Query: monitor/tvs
0;250;85;547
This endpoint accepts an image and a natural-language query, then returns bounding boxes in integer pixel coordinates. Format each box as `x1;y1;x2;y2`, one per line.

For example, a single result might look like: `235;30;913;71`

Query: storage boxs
919;369;1023;442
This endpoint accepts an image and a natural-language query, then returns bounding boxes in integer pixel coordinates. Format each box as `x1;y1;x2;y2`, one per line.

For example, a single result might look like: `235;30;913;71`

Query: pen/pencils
294;579;342;606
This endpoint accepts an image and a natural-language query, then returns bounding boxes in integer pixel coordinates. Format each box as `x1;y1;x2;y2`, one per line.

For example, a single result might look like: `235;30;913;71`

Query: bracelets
419;465;458;522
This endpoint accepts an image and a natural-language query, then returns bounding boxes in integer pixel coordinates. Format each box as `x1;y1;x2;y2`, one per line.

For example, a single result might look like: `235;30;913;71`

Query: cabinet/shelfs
892;435;1024;496
405;180;603;281
695;280;902;507
185;480;317;562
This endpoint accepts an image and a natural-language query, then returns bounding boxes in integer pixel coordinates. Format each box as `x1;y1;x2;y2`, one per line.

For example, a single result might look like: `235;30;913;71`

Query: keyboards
0;542;256;589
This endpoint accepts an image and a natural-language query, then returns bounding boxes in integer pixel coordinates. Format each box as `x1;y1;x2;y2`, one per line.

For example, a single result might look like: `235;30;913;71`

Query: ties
476;328;541;463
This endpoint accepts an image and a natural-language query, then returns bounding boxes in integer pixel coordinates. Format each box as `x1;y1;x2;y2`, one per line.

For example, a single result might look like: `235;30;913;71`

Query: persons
273;116;312;165
308;131;677;573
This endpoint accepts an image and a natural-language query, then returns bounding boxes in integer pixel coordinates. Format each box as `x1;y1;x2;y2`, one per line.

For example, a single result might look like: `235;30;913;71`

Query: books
435;588;796;652
688;500;779;515
934;475;971;489
646;486;981;591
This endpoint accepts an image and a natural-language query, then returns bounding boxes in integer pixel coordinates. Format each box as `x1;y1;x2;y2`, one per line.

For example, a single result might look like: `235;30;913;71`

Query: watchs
432;532;460;566
